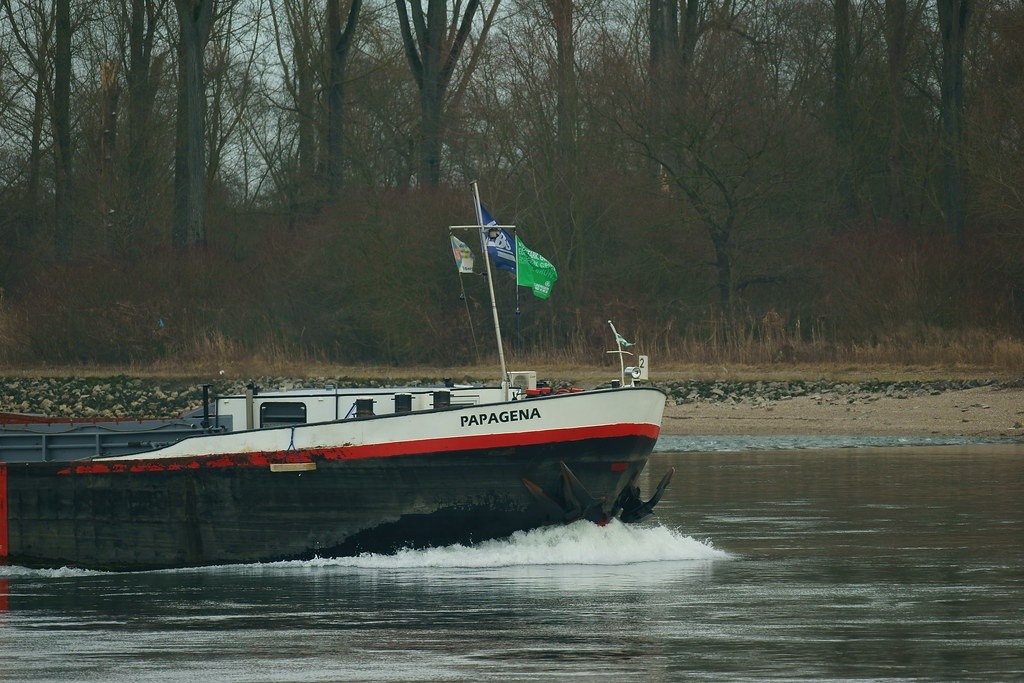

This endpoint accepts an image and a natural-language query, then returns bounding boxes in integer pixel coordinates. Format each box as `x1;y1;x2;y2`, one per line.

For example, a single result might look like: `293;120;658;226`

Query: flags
515;235;557;299
450;236;474;273
479;201;516;273
616;333;635;347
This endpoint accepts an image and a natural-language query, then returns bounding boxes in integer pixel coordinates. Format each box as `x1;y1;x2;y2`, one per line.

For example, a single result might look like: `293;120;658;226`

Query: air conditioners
508;371;537;397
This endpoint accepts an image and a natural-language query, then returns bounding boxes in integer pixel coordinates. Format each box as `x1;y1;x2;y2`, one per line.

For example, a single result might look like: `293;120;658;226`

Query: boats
0;180;677;570
